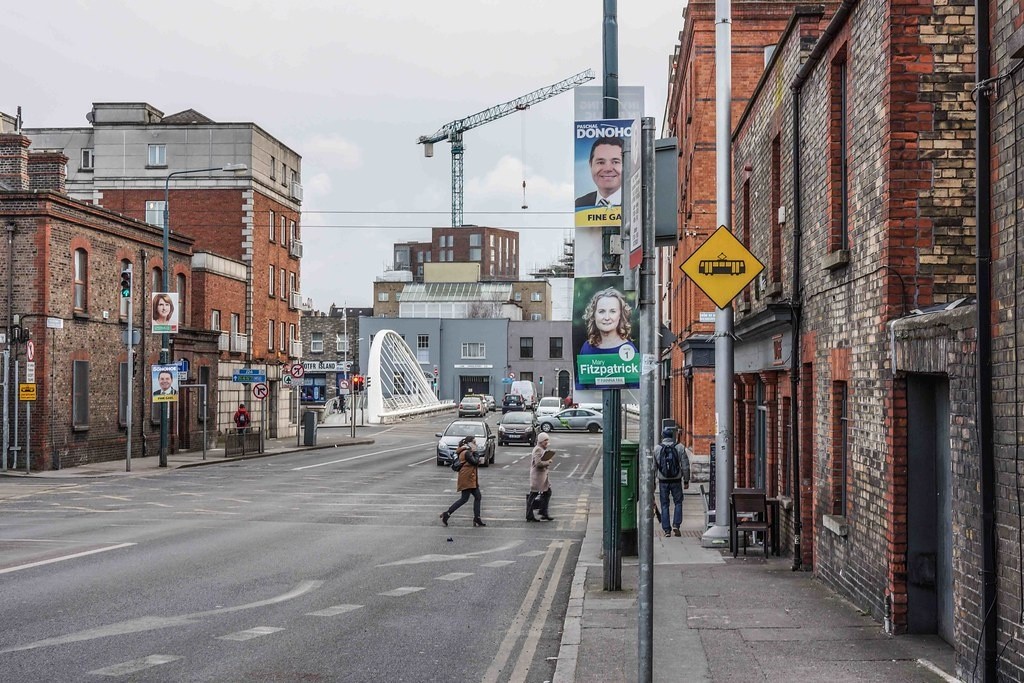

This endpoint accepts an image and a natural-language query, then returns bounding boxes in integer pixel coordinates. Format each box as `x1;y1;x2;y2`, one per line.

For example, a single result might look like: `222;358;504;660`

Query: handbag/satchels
526;493;542;509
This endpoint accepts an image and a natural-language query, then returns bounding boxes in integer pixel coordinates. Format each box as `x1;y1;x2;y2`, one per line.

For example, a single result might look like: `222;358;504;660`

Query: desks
728;496;781;557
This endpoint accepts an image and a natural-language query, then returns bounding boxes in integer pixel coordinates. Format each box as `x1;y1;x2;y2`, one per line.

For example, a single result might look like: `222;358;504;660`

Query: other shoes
673;525;681;536
665;531;671;537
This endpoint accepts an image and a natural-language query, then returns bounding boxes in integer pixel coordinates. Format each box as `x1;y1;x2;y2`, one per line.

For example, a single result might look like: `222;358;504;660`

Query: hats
662;428;673;438
538;432;549;442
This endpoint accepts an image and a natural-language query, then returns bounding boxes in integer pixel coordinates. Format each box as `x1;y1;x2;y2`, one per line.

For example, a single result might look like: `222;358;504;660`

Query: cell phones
468;442;473;447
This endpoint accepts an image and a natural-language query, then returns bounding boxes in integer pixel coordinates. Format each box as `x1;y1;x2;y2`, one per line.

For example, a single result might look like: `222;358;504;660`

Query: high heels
526;517;540;522
541;515;554;520
440;511;450;527
473;517;487;526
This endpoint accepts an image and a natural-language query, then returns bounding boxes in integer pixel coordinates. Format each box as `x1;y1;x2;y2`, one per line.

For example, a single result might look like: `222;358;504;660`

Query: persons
153;371;177;395
440;436;486;527
575;136;624;209
580;286;638;355
653;429;690;538
333;395;346;414
153;294;174;324
564;395;571;409
233;404;250;448
526;433;554;522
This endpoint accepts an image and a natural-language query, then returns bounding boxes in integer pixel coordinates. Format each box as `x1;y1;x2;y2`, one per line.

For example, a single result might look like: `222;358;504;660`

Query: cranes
417;68;597;227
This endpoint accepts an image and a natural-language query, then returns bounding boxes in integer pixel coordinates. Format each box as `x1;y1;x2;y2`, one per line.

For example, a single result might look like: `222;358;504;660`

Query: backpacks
238;412;247;423
656;442;680;478
450;457;467;471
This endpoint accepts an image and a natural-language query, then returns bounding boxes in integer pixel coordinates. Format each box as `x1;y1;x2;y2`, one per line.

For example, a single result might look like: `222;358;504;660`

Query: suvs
496;410;541;448
434;419;497;467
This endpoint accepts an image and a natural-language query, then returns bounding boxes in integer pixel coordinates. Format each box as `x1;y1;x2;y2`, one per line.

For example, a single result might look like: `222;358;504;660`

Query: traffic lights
539;377;543;385
367;376;371;387
434;378;436;386
359;377;365;391
354;377;358;390
434;368;438;374
121;271;131;297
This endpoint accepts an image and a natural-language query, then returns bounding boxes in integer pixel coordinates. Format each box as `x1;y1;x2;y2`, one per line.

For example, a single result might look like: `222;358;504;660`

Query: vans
510;380;539;412
534;396;565;426
502;393;527;415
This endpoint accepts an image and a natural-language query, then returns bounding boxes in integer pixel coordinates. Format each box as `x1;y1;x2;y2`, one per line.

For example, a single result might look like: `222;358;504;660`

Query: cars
485;395;496;412
458;394;490;417
537;408;603;434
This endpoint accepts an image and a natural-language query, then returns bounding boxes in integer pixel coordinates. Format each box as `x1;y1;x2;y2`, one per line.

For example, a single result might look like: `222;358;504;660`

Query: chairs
731;493;770;559
699;484;715;532
733;488;766;556
737;512;758;545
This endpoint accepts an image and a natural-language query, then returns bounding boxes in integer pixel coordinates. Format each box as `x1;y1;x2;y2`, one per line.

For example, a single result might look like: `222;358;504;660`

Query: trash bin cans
304;412;318;445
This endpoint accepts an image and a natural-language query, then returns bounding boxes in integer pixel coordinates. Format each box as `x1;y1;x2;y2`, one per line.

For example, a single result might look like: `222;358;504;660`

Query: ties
598;199;609;206
162;392;165;394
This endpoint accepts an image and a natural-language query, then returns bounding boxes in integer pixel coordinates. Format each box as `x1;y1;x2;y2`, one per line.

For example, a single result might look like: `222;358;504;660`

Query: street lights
159;162;248;465
